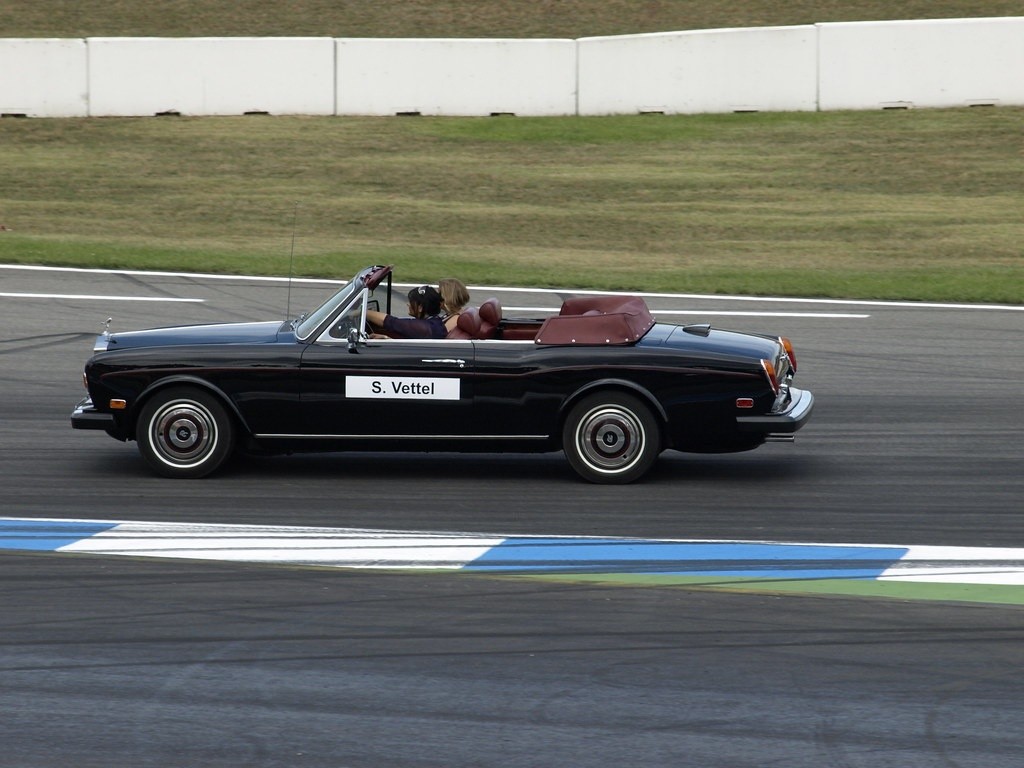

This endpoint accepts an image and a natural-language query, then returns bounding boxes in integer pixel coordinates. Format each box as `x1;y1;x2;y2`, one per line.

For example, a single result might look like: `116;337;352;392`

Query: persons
437;279;470;332
337;286;448;341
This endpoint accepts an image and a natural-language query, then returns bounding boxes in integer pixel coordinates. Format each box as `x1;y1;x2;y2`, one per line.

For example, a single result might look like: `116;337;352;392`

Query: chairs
479;298;503;338
449;307;481;340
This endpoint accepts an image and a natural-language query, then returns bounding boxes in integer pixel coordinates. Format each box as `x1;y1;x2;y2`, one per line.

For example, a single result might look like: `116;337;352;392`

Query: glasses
418;286;426;298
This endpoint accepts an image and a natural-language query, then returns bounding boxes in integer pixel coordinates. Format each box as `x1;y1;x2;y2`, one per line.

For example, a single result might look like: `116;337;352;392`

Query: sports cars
69;263;816;486
